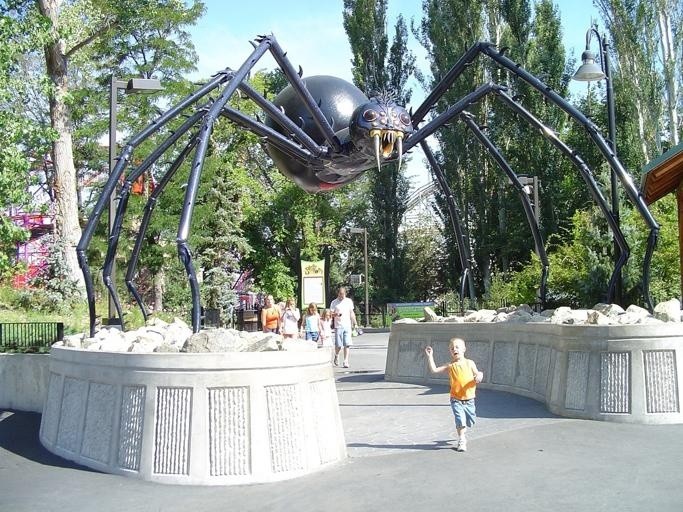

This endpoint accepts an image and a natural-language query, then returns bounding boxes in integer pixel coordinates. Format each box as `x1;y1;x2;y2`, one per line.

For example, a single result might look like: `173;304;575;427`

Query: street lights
340;225;370;328
569;24;630;310
507;173;547;310
103;72;167;333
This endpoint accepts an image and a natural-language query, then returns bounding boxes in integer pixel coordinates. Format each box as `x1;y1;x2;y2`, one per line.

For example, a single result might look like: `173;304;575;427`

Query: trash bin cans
238;310;258;332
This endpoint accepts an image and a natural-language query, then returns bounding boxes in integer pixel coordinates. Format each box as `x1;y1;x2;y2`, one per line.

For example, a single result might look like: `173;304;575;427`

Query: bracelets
353;324;357;326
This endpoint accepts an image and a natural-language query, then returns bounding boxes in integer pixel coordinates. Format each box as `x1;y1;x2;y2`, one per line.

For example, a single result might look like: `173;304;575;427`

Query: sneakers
457;441;467;452
334;356;349;368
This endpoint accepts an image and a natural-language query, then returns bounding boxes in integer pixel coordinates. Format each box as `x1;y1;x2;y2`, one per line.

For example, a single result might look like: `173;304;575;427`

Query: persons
299;301;321;343
330;287;359;368
254;294;300;341
320;308;334;347
424;337;483;452
